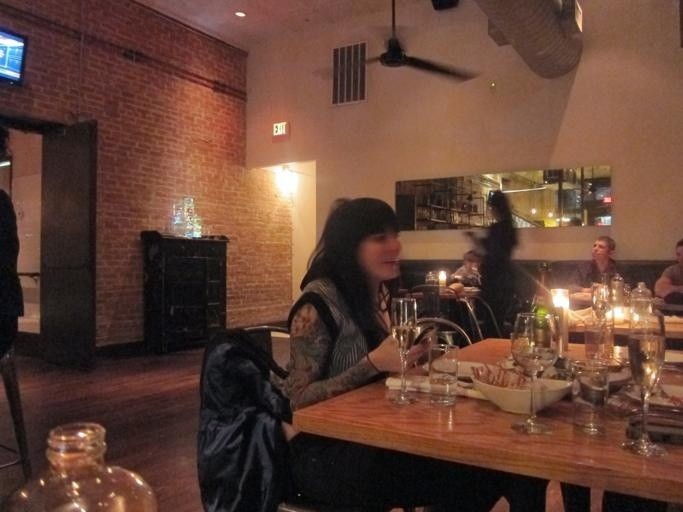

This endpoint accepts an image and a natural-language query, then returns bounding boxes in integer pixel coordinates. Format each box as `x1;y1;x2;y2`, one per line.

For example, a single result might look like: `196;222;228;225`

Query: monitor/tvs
0;27;28;86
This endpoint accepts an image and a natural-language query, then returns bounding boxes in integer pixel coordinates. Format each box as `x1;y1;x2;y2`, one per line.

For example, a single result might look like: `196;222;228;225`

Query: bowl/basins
472;377;573;417
464;290;480;296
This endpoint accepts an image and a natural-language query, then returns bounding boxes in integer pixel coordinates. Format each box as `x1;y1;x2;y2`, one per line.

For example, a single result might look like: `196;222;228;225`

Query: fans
311;1;481;87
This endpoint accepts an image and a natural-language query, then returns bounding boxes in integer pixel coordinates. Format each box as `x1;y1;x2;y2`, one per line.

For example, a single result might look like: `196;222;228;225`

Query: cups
592;282;610;324
427;341;461;411
583;310;617;367
570;357;610;439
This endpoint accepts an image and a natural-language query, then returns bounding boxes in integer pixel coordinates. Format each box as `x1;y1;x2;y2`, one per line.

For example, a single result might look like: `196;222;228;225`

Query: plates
619;347;683;366
622;384;683;414
421;359;500;383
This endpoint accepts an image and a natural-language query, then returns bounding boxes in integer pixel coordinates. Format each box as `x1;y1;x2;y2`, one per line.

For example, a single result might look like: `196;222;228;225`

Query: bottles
531;259;557;353
611;272;656;321
3;421;159;511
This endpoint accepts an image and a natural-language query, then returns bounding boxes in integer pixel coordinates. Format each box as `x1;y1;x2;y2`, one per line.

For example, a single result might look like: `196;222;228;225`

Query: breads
447;282;465;295
470;364;525;389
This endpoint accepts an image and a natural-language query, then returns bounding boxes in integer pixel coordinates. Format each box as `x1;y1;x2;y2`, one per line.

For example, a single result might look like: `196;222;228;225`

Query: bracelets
365;353;384;376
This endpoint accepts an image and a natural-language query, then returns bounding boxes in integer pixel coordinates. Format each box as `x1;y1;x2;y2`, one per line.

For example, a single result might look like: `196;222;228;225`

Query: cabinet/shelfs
140;230;229;358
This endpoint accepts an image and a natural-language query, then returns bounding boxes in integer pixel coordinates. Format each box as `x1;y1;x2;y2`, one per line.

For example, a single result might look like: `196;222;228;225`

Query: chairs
199;326;448;512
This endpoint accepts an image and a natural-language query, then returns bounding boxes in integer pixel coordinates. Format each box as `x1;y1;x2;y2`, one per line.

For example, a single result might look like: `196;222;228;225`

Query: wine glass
388;296;419;407
509;312;563;436
620;309;670;461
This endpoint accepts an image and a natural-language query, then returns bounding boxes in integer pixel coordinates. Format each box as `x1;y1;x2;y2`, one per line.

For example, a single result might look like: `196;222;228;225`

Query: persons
653;239;682;298
282;193;519;510
566;236;622;309
450;248;482;287
464;190;521;338
